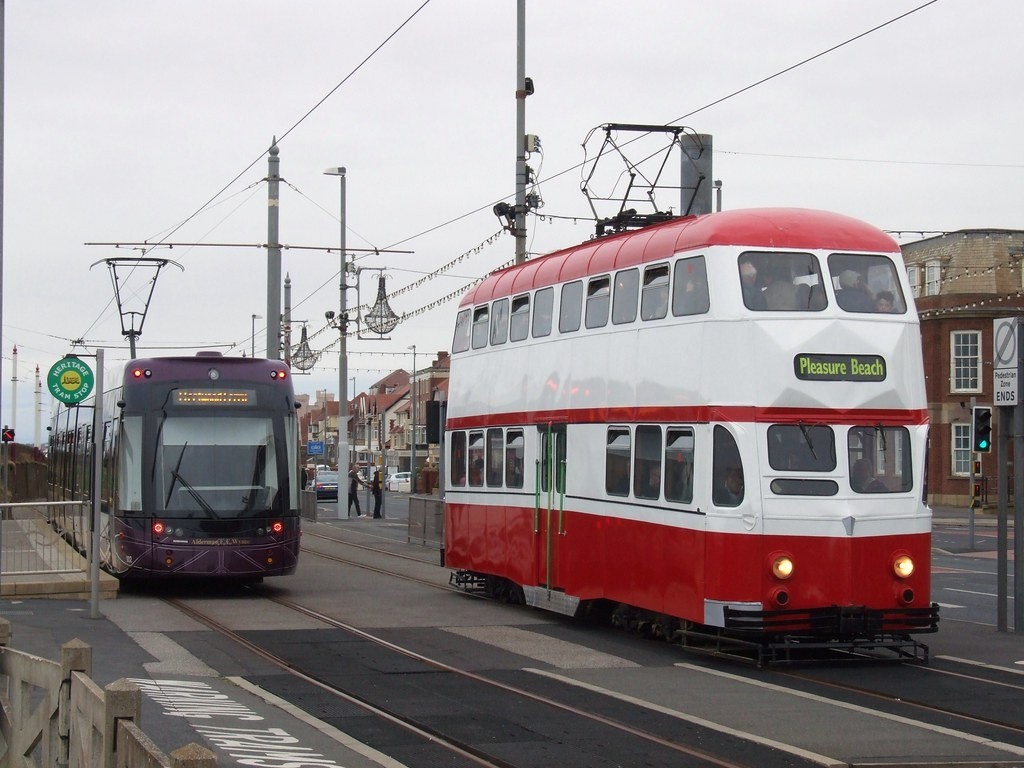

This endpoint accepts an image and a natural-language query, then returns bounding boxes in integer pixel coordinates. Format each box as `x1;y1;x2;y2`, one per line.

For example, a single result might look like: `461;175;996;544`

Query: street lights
406;343;417;497
323;165;351;522
322;388;330;469
350;377;355;465
251;314;263;358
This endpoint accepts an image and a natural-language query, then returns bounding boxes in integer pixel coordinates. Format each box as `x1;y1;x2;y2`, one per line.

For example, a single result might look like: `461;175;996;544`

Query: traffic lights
975;408;992;452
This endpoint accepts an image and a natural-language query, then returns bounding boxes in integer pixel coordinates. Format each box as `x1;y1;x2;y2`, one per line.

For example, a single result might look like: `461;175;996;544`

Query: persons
474;453;485;484
851;458;890;493
301;466;307;491
741;260;767;310
371;466;382;519
833;269;899;314
348;464;374;518
711;460;744;505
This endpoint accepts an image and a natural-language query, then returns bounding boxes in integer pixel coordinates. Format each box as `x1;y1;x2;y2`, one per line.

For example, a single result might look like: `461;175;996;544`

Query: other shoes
358;514;367;518
348;516;352;518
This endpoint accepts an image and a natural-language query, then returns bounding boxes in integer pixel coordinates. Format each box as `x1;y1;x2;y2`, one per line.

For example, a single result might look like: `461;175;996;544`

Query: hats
839;269;861;288
852;459;869;482
645;445;667;466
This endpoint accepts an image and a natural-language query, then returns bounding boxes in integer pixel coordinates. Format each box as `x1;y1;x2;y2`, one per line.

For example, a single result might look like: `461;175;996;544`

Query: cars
386;471;412;491
316;464;332;471
316;471;338;498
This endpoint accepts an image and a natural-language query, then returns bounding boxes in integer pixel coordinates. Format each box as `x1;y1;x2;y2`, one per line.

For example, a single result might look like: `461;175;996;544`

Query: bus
441;206;942;663
47;350;304;589
